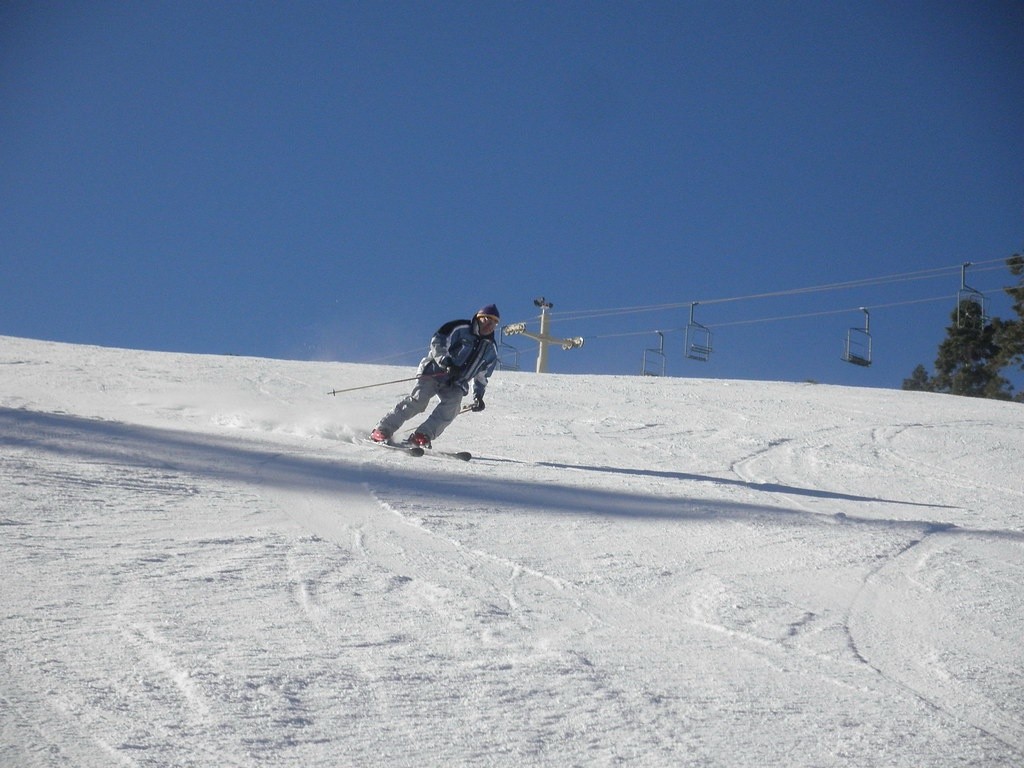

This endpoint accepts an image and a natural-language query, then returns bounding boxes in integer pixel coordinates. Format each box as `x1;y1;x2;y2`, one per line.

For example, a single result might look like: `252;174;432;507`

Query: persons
368;304;500;451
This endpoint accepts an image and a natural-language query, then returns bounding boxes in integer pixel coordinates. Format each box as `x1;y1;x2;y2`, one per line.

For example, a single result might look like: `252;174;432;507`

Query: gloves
471;398;485;412
446;364;461;382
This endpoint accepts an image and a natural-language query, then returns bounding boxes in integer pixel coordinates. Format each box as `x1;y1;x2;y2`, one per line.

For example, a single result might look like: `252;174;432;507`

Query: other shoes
372;429;394;445
411;432;432;450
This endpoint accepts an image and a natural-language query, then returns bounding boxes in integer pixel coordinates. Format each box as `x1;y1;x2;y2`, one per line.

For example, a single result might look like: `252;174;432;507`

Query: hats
476;304;499;322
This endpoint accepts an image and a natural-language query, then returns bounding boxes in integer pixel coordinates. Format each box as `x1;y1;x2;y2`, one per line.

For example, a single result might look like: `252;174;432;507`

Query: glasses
485;317;498;330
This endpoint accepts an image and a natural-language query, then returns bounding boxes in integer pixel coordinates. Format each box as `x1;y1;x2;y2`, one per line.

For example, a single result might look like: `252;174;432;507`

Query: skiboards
357;437;471;461
